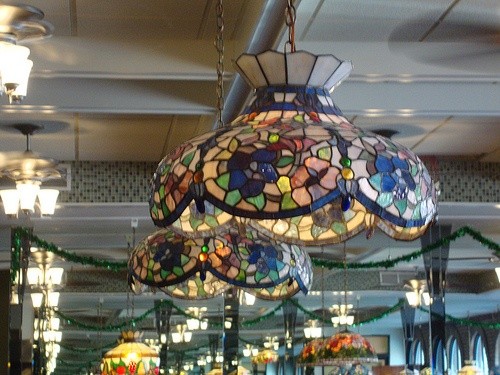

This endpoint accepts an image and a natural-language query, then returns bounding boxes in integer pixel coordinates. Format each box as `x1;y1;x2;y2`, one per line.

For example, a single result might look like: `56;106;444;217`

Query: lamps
403;278;432;307
128;228;316;300
148;0;439;247
0;122;61;218
303;319;322;338
0;0;56;105
331;304;355;325
